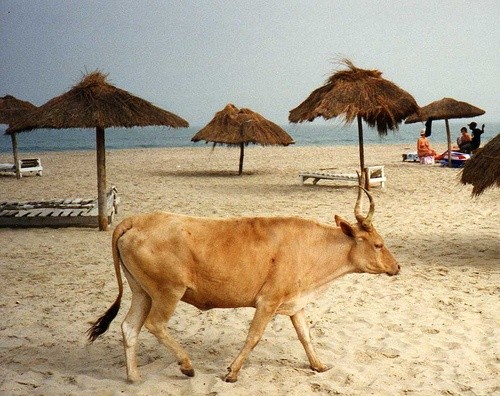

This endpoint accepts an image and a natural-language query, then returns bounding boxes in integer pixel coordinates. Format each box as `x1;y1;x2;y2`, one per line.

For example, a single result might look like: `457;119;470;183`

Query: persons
456;126;472;154
417;128;437;158
460;122;485;154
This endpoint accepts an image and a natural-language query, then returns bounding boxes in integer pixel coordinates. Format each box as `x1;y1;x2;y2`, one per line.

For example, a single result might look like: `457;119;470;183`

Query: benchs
0;156;44;180
401;151;449;162
0;186;121;228
299;165;386;189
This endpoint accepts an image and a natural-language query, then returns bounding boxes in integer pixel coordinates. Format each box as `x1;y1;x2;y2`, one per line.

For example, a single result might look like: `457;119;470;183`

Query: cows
86;170;401;384
